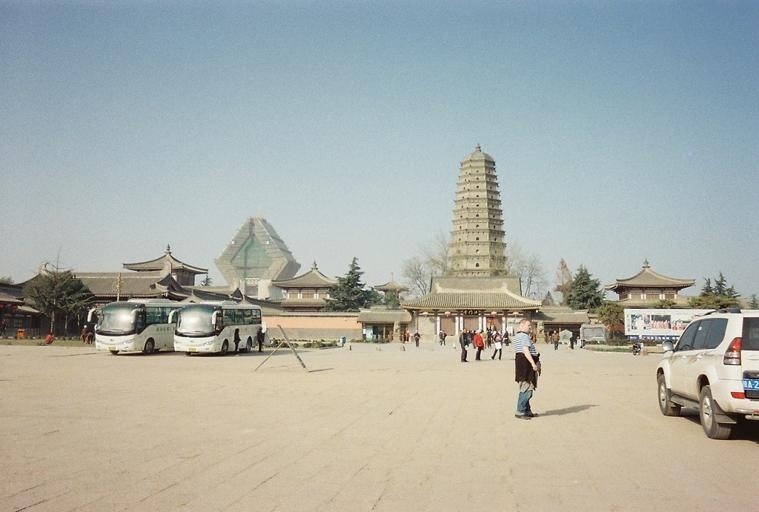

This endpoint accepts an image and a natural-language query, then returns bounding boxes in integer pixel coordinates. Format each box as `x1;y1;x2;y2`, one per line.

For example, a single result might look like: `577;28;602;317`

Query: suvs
652;303;759;438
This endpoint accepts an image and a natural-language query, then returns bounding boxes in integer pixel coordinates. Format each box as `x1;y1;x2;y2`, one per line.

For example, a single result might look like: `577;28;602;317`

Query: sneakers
526;413;538;417
515;415;530;420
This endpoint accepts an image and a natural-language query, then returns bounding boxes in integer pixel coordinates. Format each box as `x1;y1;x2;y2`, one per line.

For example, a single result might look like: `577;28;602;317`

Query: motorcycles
631;341;641;356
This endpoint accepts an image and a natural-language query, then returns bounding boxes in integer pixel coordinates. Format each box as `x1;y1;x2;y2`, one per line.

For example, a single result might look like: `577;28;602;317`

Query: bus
167;301;264;355
86;298;181;354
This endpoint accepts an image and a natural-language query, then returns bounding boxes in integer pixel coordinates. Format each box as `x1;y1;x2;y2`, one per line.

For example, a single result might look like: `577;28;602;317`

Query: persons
342;317;585;421
44;332;57;344
235;328;241;355
257;326;264;352
81;324;94;344
